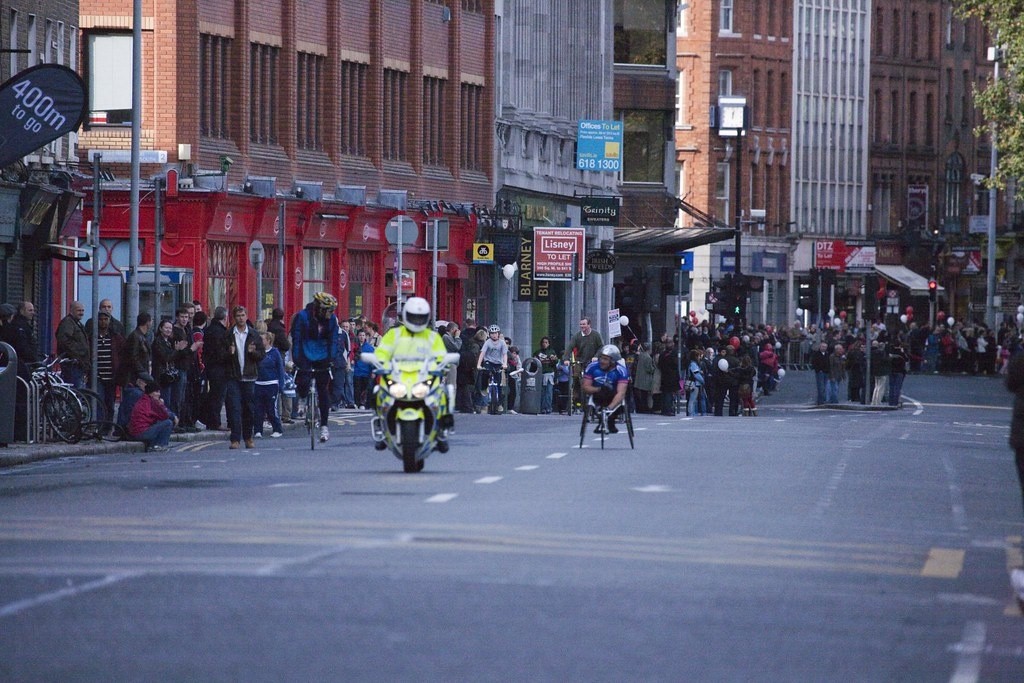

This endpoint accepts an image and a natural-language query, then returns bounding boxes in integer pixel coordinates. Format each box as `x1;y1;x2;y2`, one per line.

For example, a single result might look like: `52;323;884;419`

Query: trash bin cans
0;342;17;443
519;358;543;415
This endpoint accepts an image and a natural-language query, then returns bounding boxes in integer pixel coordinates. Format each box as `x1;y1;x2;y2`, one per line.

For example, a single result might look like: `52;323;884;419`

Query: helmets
598;344;621;363
402;296;431;332
312;291;338;312
487;325;501;333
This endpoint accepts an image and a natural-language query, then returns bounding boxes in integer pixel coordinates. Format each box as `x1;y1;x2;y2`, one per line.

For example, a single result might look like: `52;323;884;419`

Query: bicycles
25;354;124;445
293;364;334;450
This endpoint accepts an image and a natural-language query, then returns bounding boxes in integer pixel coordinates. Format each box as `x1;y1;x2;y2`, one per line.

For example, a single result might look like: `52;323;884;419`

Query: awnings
874;265;946;291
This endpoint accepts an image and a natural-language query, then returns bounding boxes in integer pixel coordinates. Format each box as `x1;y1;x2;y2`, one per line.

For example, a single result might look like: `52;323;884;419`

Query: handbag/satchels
160;360;179;382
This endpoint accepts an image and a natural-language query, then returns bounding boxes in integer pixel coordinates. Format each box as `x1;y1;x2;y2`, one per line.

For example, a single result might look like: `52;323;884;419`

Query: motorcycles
360;353;460;472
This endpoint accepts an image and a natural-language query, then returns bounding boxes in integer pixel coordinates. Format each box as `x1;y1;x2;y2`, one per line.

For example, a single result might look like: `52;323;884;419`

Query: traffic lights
799;274;818;312
731;304;744;319
929;281;936;302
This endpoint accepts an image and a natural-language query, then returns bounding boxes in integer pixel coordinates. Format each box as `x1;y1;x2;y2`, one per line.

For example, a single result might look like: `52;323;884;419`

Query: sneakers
254;432;263;438
320;426;329;441
375;437;388;450
244;438;255;448
270;432;283;438
436;436;449;454
228;441;241;449
498;404;504;411
205;424;231;431
506;410;517;414
481;389;488;396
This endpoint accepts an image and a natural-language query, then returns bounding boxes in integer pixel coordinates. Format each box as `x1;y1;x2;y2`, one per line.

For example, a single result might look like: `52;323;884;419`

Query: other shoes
175;424;196;433
146;446;154;451
328;399;378;413
151;444;169;451
284;420;295;424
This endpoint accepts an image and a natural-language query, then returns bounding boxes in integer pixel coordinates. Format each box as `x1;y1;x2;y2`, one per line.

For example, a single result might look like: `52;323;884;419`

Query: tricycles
579;395;636;450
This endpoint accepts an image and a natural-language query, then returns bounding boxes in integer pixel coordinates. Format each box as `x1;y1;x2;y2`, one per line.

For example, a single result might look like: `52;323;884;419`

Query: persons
217;305;266;449
438;317;522;415
116;372;179;433
1005;328;1024;510
0;301;35;440
292;291;339;443
532;317;605;415
253;308;300;438
127;300;234;434
127;382;173;453
790;297;1020;407
331;315;383;412
620;313;790;416
373;296;450;453
55;299;129;438
581;344;633;434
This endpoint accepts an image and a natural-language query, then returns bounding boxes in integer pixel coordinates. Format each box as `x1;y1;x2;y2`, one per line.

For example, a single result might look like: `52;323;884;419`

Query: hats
145;383;161;394
